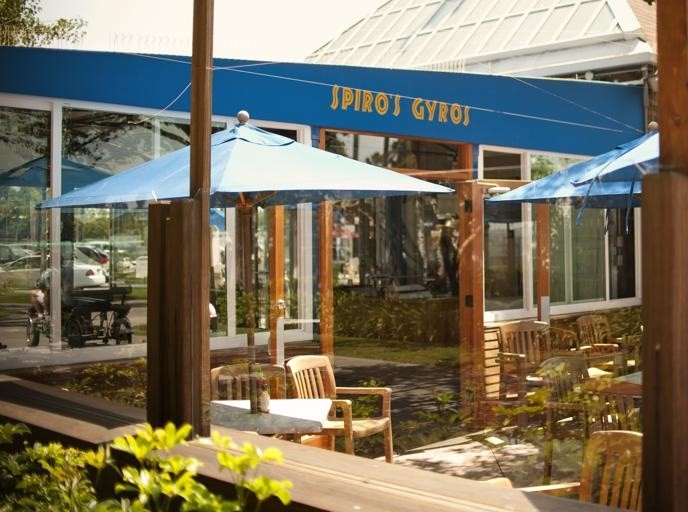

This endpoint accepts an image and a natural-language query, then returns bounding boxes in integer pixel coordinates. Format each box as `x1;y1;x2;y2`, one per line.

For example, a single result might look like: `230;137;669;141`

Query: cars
0;240;137;289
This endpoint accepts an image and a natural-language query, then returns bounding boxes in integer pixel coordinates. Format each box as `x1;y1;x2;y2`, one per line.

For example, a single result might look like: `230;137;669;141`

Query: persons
30;254;66;323
425;235;459;298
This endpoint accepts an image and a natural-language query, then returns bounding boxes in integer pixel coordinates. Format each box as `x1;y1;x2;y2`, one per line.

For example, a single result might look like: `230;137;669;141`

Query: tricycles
27;285;134;348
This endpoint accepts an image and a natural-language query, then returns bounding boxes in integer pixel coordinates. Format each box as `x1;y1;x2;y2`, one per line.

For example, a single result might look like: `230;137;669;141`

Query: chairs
286;352;393;468
212;355;327;454
495;310;645;511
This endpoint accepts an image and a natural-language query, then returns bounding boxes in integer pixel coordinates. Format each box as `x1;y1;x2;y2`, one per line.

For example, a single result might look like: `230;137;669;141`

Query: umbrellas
484;121;660;235
34;109;455;415
0;150;224;233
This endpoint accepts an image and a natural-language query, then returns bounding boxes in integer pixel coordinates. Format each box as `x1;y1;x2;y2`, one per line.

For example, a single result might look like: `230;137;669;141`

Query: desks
212;398;334;443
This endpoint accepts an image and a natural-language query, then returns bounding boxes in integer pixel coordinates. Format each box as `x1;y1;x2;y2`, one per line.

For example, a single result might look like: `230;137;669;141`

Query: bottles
256;377;270;413
